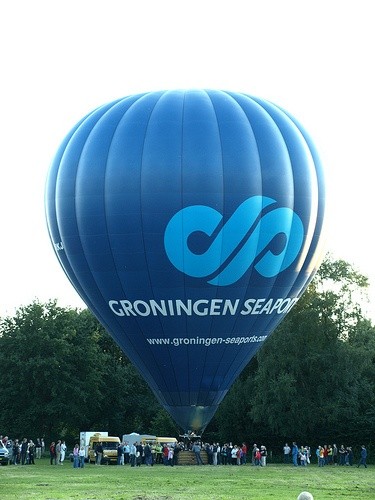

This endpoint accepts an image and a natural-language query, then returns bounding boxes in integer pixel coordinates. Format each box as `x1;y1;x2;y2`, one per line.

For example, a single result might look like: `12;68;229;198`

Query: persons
0;435;46;465
298;491;313;500
356;445;367;468
284;442;312;466
252;444;268;467
339;445;354;466
179;440;247;466
73;444;86;468
316;444;338;467
94;442;103;466
117;441;180;467
50;440;67;465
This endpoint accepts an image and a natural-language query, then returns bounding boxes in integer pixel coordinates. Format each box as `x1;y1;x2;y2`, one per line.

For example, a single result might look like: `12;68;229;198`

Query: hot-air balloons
43;88;331;465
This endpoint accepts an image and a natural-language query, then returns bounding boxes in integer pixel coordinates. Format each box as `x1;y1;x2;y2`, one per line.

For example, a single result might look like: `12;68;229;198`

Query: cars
0;441;12;465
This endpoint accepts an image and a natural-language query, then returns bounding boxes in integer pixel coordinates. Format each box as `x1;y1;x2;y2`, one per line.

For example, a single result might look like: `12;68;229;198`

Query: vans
142;437;179;450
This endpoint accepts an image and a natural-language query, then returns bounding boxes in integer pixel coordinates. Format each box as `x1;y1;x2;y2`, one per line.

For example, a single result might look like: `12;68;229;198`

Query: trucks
79;431;122;465
